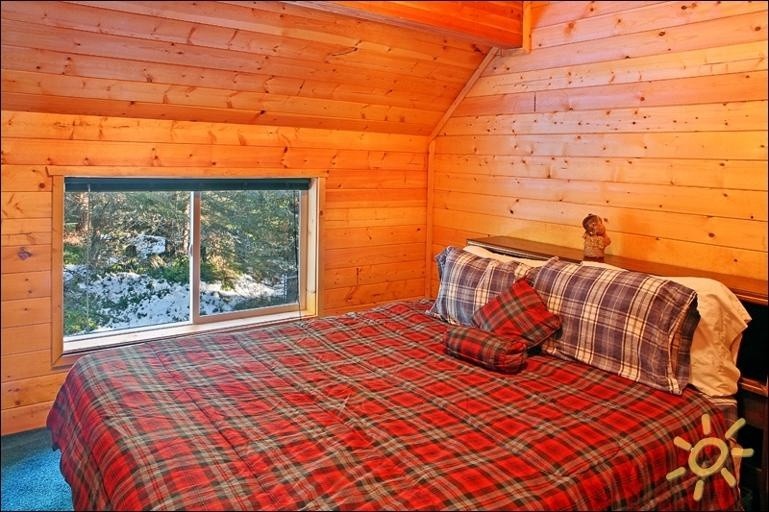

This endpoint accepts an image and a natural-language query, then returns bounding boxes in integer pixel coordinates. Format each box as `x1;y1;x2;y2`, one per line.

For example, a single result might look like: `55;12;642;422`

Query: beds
70;235;743;512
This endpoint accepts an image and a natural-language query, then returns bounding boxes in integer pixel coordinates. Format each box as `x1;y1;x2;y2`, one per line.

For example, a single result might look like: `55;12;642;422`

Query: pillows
467;277;562;350
425;247;534;328
533;255;699;396
442;326;527;372
